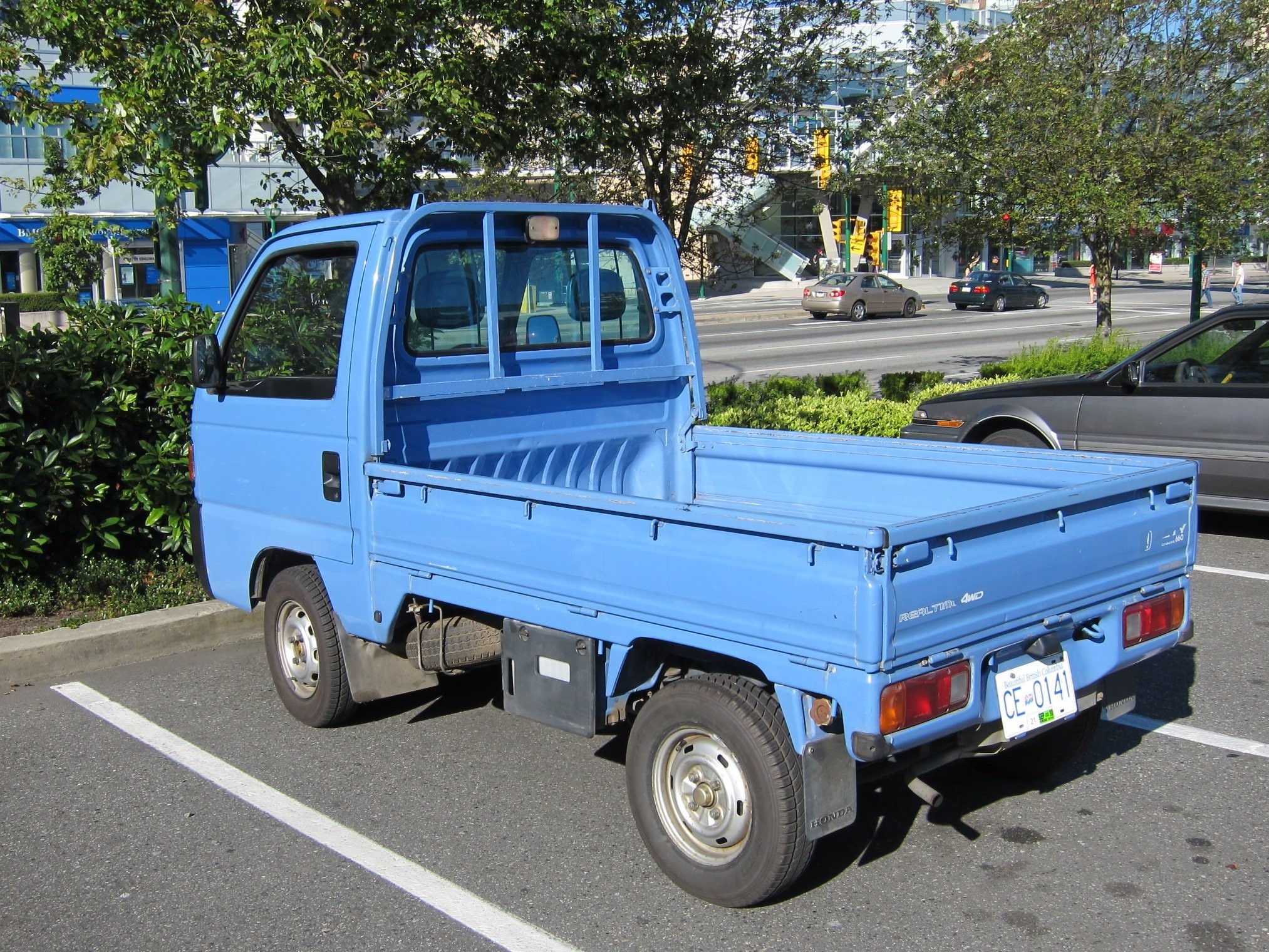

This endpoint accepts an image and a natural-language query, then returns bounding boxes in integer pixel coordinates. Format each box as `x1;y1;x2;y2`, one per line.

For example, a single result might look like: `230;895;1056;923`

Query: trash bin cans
0;302;21;340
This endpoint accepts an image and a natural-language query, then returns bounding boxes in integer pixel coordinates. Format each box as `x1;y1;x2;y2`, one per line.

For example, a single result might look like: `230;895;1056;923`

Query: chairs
866;280;874;288
415;272;487;355
568;271;626;340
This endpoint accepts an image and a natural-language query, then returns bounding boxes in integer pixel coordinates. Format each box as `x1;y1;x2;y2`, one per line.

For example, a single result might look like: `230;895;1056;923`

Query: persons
1086;259;1097;304
1201;260;1213;308
965;250;980;276
988;256;1000;271
1231;260;1244;305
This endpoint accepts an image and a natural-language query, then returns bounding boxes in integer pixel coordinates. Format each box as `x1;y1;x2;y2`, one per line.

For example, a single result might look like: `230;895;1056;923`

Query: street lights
831;90;850;273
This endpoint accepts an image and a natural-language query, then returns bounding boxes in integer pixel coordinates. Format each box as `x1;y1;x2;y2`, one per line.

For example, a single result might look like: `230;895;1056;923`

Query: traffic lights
866;231;881;265
855;219;866;241
831;219;842;243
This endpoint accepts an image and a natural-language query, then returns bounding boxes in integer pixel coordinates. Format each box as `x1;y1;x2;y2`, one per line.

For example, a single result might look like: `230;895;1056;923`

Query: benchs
827;278;851;286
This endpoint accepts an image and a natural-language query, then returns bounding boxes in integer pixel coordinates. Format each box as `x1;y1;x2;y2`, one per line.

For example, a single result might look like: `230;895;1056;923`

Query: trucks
188;197;1199;910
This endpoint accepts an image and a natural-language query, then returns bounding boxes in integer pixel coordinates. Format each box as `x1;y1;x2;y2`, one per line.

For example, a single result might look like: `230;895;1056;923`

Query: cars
947;269;1050;312
801;272;926;322
897;298;1269;515
